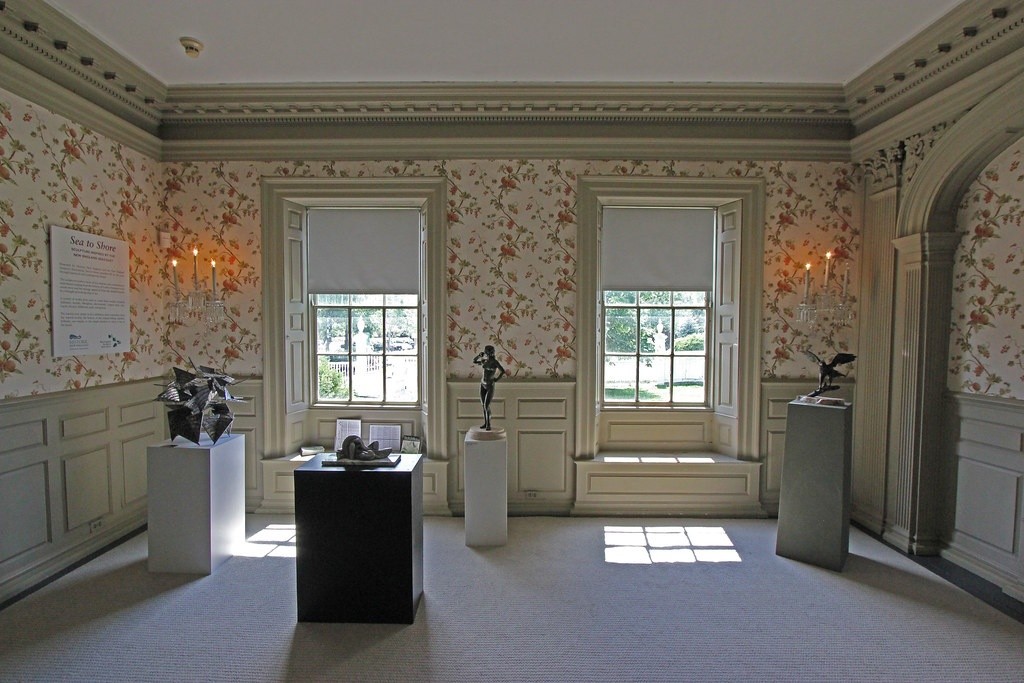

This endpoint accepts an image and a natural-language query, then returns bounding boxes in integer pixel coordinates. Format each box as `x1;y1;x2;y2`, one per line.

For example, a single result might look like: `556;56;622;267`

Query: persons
473;345;506;431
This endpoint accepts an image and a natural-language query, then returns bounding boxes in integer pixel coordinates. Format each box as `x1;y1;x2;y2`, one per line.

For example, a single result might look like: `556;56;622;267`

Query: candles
172;260;181;292
841;262;850;295
804;263;811;297
823;251;831;286
210;258;218;294
192;246;199;288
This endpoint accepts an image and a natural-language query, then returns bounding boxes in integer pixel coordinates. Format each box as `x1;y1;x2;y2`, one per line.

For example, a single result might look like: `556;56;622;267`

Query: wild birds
799;350;858;390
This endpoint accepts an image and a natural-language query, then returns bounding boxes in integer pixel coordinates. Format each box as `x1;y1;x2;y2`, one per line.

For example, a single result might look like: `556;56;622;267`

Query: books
334;418;361;452
369;425;420;454
300;445;325;456
